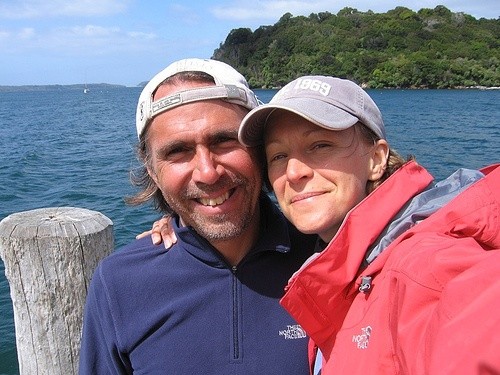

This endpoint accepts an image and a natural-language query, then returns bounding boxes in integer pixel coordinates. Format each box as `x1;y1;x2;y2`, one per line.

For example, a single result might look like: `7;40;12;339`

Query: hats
136;58;260;142
238;76;386;148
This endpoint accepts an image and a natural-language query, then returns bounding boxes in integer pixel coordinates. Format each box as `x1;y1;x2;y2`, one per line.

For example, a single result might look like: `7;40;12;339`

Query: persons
78;59;328;375
134;75;500;375
